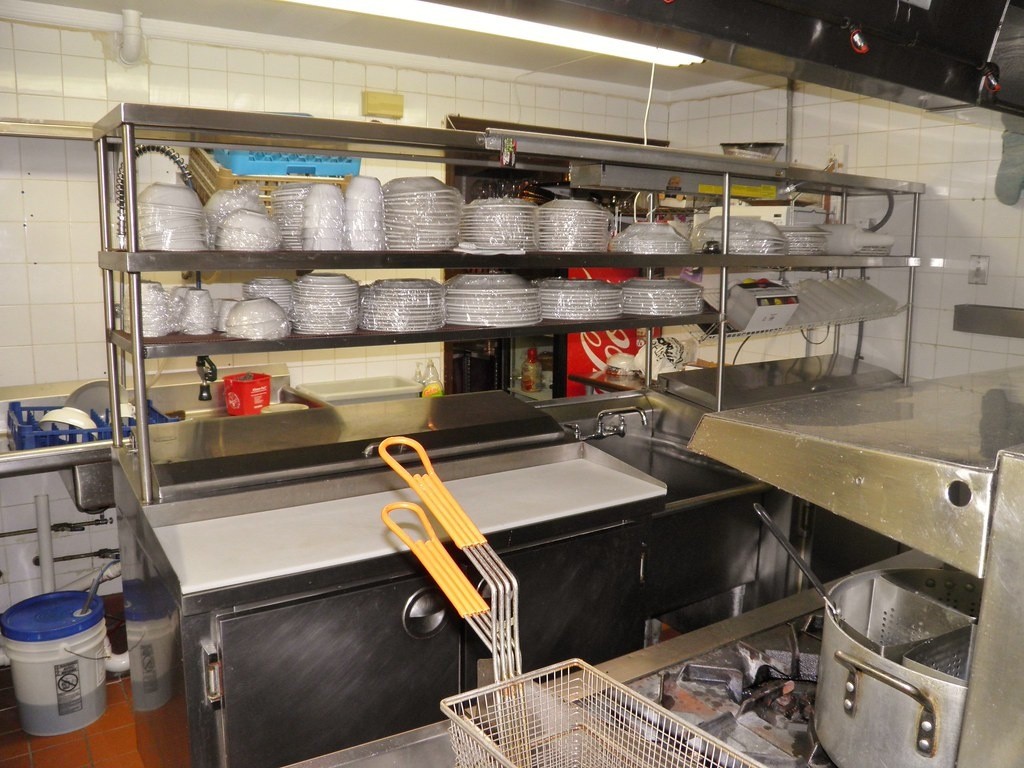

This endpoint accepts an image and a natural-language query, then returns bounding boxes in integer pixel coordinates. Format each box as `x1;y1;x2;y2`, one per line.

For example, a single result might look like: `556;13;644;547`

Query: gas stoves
573;603;841;768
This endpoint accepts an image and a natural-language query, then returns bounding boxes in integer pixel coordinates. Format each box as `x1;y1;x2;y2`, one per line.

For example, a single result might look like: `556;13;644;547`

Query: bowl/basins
38;407;98;442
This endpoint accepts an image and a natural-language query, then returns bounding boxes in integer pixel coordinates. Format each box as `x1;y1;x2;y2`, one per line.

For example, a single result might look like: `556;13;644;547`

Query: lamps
280;1;706;69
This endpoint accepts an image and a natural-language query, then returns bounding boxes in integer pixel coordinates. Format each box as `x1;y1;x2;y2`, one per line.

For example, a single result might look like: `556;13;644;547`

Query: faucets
604;401;650;439
195;382;218;404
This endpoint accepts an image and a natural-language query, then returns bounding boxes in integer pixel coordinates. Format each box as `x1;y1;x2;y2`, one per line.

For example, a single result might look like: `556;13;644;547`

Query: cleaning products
420;358;449;398
413;360;424;398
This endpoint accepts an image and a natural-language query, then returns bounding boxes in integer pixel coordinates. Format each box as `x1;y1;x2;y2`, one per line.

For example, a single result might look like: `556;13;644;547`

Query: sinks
590;434;769;628
9;380;337;519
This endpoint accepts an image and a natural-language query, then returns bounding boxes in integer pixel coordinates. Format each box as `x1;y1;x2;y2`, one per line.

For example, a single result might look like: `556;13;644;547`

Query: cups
119;400;136;425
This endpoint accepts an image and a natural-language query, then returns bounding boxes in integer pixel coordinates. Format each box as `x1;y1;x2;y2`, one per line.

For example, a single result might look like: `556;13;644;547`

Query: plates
119;175;898;339
64;378;129;417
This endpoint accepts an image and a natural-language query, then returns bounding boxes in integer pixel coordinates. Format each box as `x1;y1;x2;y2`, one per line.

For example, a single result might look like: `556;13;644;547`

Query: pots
752;500;987;768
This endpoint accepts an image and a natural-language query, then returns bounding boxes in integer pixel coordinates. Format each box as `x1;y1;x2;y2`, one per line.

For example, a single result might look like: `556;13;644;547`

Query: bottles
420;359;444;398
520;348;542;392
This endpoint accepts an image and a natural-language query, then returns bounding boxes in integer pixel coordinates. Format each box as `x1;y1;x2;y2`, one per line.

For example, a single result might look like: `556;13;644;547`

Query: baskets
439;656;767;768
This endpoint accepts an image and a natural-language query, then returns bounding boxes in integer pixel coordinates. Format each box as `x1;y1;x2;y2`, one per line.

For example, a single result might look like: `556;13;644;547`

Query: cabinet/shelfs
90;100;928;503
114;489;911;768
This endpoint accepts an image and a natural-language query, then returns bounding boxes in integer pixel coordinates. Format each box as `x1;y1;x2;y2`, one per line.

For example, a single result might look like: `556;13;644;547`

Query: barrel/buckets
220;372;271;416
0;590;107;738
119;574;179;714
